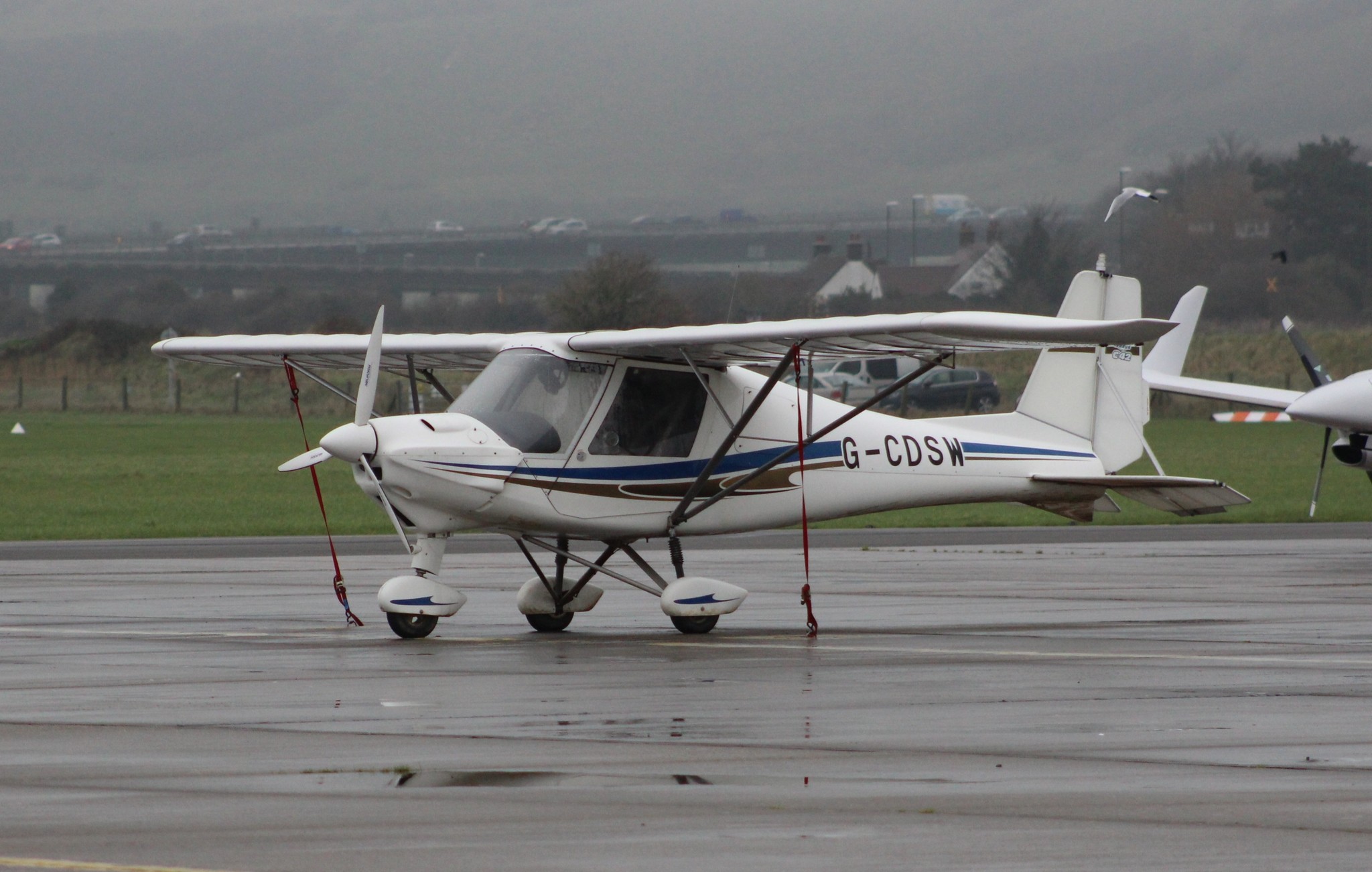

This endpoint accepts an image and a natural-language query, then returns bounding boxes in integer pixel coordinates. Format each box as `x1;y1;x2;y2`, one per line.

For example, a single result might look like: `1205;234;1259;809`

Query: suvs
828;353;921;388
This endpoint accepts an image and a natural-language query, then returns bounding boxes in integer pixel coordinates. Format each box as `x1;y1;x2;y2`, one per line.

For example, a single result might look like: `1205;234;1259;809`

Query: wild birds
1104;186;1159;222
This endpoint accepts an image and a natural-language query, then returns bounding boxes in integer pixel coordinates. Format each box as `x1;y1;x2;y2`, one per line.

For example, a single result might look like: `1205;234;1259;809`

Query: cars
876;365;1002;415
783;372;875;409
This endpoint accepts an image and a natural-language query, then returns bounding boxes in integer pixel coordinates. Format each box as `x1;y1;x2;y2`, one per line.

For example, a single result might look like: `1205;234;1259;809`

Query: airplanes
152;256;1252;638
1143;284;1371;517
